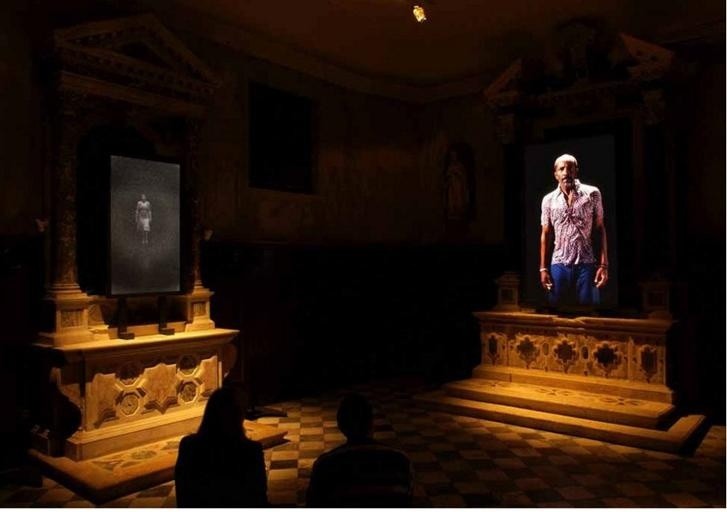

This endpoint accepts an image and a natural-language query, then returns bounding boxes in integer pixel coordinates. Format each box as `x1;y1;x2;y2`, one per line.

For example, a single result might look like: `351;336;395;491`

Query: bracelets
600;263;609;269
538;267;548;271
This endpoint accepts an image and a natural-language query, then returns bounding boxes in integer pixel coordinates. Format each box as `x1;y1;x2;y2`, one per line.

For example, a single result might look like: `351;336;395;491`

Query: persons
306;394;414;506
172;383;270;507
135;194;152;244
536;151;609;314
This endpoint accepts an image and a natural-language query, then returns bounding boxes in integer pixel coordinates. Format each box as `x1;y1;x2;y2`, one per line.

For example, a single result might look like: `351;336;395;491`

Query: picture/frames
96;144;193;302
